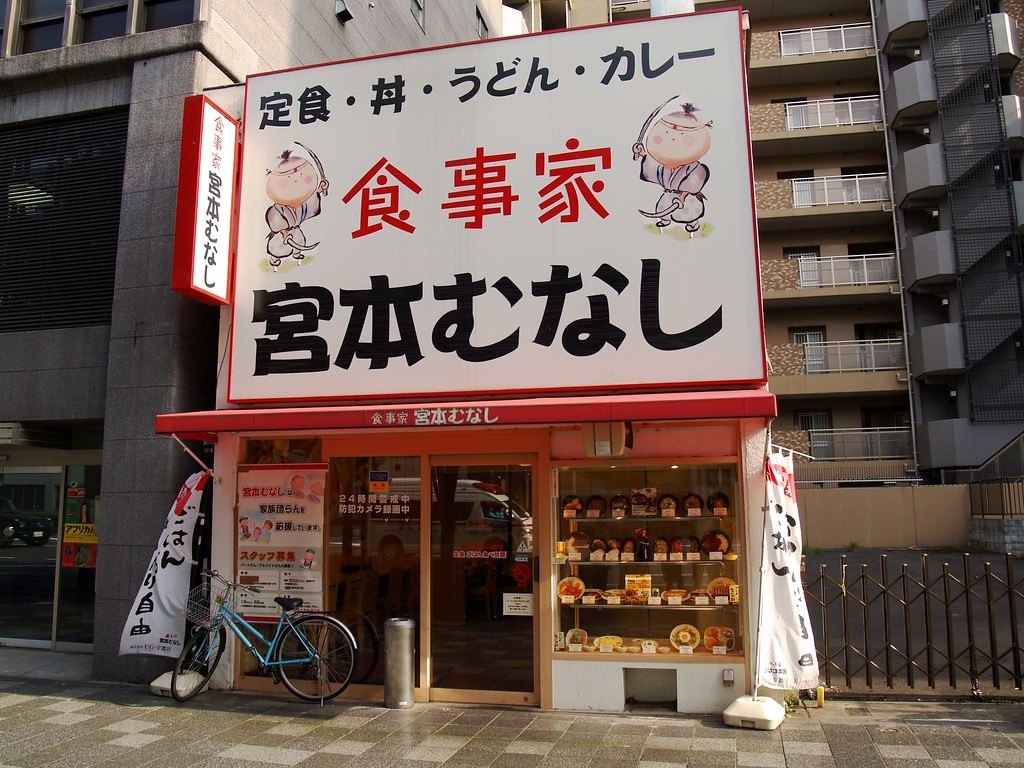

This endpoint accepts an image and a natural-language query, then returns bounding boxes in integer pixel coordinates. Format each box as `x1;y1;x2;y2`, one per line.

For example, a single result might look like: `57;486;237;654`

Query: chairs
377;563;421;621
330;571;381;664
473;567;500;623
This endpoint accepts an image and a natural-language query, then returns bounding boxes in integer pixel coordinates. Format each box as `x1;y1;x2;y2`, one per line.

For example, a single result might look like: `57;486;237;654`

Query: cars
0;496;51;549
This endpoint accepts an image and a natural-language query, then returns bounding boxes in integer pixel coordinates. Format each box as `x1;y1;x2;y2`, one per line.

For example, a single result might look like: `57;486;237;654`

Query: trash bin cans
381;616;416;710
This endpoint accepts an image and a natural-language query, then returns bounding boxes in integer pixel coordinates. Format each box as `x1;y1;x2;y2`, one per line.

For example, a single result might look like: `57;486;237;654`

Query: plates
585;495;607;516
590;537;636;556
557;577;585;601
706;492;730;512
593;636;623;648
609;495;632;518
657;494;679;513
642;640;658;651
601;589;625;602
681;493;704;513
662;589;690;601
562;496;583;515
566;629;587;647
708;577;736;601
582;588;603;600
670;624;700;650
653;536;701;555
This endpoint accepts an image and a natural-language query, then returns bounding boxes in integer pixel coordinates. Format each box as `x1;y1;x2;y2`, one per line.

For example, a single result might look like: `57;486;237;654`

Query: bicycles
169;568;358;712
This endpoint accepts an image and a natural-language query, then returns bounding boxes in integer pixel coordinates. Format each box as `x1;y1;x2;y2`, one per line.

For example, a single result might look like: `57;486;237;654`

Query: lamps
914;50;921;57
950;391;957;397
942;299;948;305
921;128;930;137
932;211;939;216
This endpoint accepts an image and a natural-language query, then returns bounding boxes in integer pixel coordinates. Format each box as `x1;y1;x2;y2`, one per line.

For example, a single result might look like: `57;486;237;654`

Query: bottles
702;530;732;555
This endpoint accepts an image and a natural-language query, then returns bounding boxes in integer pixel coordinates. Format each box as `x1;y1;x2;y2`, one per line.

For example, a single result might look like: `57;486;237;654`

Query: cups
652;588;659;597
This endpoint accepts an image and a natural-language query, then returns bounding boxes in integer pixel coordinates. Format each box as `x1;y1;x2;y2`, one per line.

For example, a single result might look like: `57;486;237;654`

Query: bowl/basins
585;646;595;652
629;646;641;652
658;647;670;654
617;646;628;653
632;494;647;505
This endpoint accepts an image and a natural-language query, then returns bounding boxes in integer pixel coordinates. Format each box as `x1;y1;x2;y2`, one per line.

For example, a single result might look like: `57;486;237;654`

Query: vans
338;475;534;587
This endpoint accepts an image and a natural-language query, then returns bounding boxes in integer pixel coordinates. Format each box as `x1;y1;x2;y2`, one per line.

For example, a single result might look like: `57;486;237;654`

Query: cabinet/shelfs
553;460;745;659
